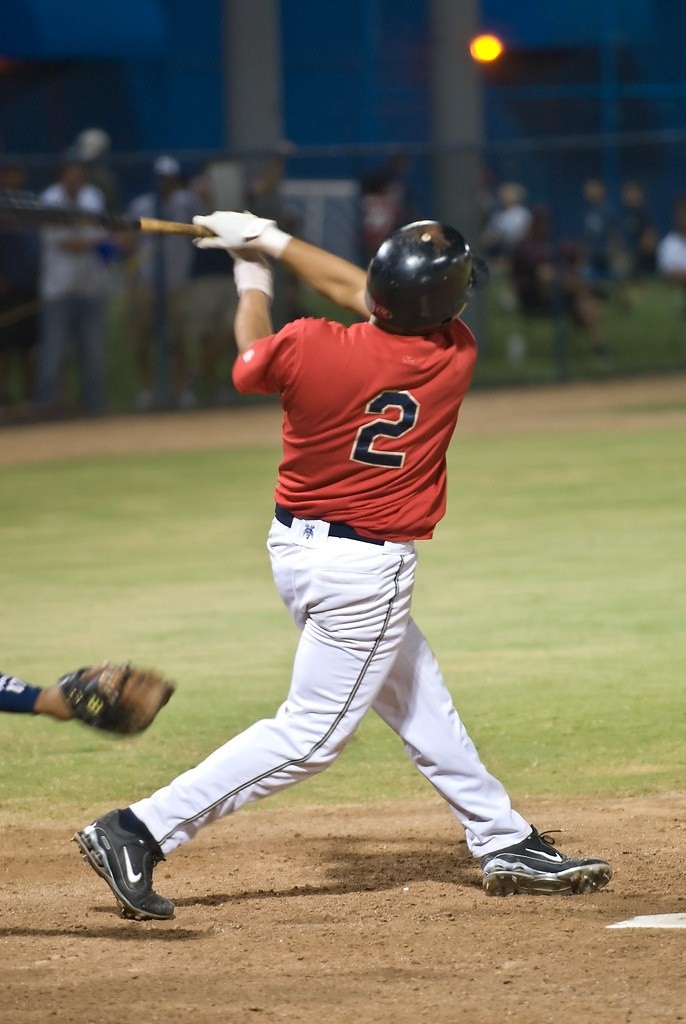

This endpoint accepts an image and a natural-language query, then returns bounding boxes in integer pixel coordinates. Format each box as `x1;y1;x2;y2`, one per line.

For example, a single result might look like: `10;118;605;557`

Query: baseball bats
1;195;216;237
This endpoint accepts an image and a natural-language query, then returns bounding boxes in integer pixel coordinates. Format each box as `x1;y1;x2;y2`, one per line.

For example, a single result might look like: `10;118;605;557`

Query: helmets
364;221;490;332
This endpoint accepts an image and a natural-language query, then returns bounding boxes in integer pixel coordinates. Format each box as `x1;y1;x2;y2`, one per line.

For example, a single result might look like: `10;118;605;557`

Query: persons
71;212;611;921
0;659;109;722
0;153;686;414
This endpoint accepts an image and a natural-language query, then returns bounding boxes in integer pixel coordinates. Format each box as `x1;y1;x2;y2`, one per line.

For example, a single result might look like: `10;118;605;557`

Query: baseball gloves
57;662;174;739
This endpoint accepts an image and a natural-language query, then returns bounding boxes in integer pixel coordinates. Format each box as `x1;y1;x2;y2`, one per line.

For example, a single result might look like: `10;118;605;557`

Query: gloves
192;210;293;259
224;246;275;301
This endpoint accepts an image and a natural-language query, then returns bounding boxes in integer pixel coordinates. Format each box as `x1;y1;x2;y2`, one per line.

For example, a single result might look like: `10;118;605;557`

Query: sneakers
70;808;174;921
481;824;613;897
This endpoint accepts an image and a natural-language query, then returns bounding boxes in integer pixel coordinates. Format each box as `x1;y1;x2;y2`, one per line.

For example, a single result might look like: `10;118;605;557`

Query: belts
275;502;386;546
